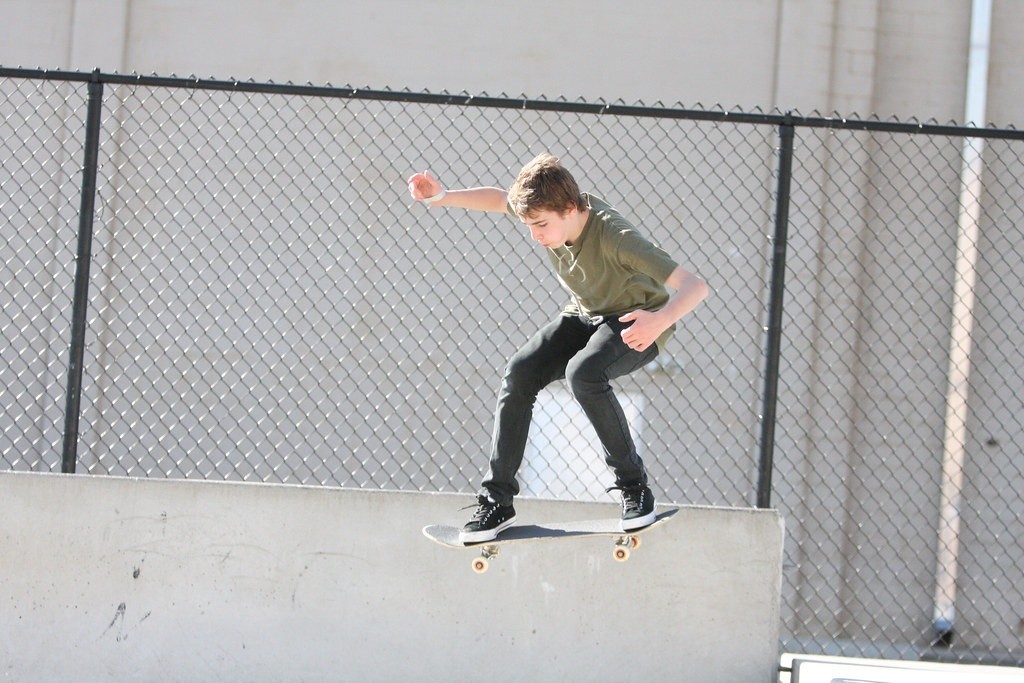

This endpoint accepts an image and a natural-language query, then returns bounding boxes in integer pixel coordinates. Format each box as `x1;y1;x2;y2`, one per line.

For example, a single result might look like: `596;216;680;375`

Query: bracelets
422;189;446;204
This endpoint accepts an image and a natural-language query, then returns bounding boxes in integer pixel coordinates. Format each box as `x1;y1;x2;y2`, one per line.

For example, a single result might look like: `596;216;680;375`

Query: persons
407;153;706;544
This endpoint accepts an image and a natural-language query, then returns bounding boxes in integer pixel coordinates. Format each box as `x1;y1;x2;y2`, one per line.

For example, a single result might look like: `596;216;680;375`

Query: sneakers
457;495;517;542
606;482;656;530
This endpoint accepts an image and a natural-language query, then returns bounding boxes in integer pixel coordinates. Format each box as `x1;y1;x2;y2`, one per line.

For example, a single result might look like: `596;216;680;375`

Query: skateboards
422;507;682;574
